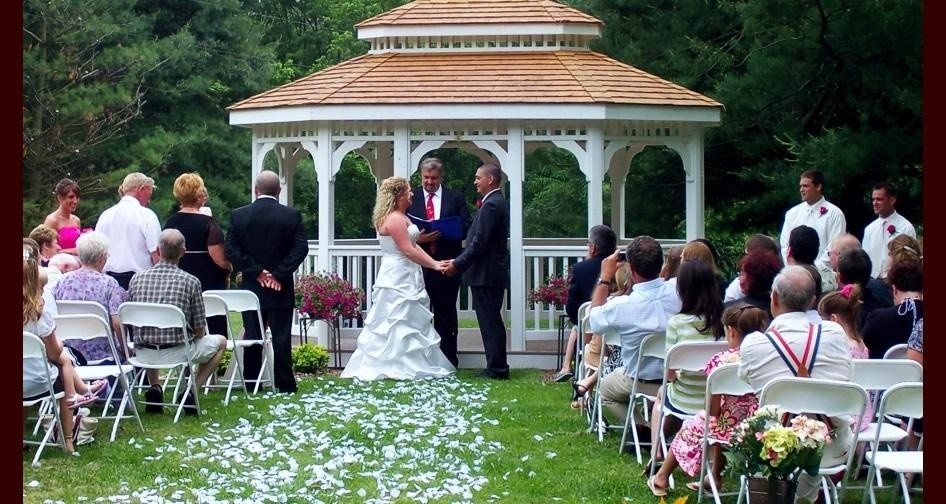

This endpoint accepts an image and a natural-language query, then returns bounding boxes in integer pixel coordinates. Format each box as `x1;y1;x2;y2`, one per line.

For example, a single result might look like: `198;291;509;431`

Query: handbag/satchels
41;415;98;445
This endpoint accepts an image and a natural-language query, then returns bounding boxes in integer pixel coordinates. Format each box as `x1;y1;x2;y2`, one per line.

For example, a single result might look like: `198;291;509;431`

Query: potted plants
291;345;329;378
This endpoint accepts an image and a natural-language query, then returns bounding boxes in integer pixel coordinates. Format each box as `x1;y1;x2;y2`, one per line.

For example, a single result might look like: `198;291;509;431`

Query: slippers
686;482;713;497
647;475;667;496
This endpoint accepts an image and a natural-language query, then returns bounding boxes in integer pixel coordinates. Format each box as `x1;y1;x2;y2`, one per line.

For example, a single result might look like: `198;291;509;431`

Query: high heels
64;436;80;457
554;370;589;410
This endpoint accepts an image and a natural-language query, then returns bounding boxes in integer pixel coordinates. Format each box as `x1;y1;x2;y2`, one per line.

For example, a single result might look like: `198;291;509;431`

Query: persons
554;222;925;503
861;183;917;283
163;172;233;376
224;169;309;393
24;224;230;459
780;169;847;293
408;158;473;369
339;175;456;380
95;172;161;291
44;178;81;256
442;163;509;380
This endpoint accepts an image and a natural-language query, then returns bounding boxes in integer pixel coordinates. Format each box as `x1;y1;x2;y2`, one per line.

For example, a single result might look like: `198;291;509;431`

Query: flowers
293;269;362;327
527;268;574;307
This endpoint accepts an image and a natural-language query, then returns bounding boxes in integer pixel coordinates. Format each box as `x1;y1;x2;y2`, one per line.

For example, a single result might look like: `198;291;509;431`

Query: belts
139;342;179;349
631;377;663;384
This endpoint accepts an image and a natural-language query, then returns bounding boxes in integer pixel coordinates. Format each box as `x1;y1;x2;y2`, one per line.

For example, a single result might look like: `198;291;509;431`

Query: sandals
65;393;99;410
85;379;108;396
146;384;163;413
178;390;197;414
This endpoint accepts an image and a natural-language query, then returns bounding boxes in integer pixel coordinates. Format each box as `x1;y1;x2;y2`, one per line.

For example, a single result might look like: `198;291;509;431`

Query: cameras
616;253;627;262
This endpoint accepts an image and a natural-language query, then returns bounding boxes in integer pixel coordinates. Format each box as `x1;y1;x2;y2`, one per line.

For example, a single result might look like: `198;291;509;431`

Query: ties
805;208;814;227
871;220;886;279
426;193;435;254
